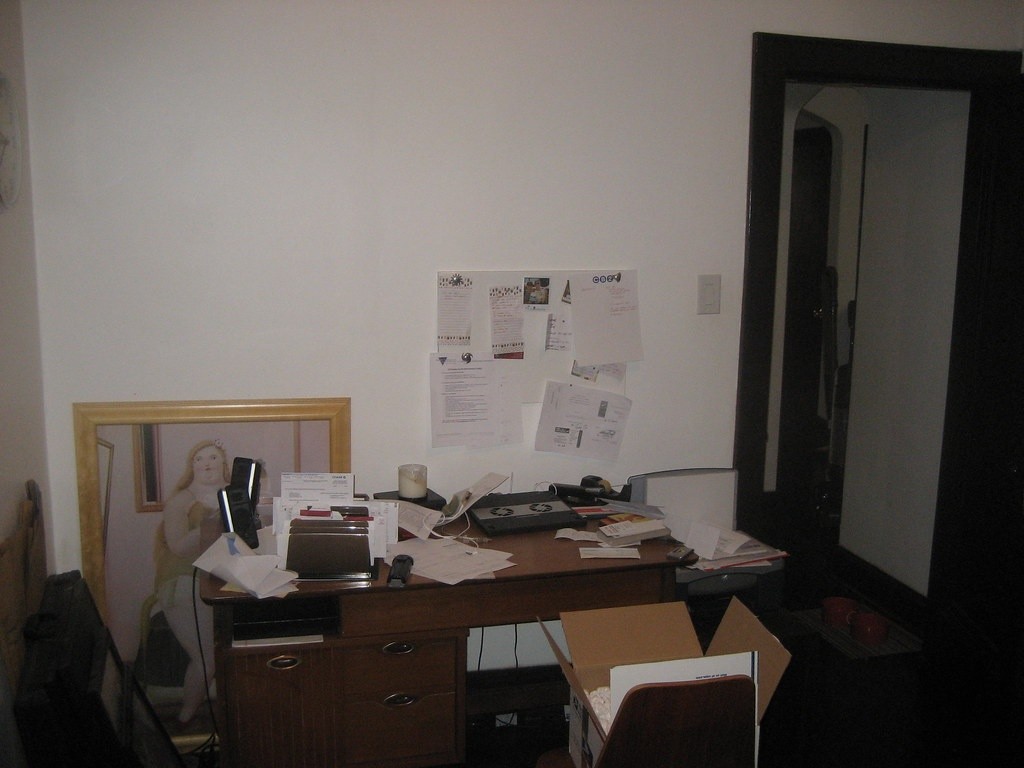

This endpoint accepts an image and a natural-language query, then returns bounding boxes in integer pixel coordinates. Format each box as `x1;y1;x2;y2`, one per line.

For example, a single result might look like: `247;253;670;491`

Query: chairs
596;674;757;768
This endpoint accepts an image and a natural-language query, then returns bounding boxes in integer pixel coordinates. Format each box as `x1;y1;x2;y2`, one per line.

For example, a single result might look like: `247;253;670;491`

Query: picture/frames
74;396;353;753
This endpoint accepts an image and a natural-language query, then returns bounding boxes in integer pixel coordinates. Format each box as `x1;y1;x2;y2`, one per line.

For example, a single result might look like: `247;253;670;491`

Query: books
597;521;672;545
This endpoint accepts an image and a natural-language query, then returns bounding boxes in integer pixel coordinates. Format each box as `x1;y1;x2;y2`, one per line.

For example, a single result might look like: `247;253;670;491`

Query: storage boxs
535;595;794;768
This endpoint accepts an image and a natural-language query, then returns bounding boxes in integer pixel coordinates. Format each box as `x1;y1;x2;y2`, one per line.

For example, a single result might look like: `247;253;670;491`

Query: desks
195;500;700;768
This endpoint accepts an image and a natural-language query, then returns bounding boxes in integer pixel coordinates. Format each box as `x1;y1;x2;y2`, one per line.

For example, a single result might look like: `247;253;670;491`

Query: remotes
387;554;414;588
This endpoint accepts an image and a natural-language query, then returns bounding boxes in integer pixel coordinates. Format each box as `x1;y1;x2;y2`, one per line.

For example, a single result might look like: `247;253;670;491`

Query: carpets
791;603;922;660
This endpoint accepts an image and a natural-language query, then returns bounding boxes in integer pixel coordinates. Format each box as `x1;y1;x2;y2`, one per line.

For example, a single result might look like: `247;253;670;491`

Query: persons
525;279;549;303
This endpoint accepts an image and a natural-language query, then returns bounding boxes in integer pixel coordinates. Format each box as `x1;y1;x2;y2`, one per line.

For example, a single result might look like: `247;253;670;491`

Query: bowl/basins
822;595;891;643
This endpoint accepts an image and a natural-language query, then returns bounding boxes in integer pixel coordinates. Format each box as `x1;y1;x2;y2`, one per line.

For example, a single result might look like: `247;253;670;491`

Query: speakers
217;456;262;555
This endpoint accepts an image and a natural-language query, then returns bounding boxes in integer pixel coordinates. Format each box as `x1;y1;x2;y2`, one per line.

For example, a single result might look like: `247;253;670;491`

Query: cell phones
665;546;695;561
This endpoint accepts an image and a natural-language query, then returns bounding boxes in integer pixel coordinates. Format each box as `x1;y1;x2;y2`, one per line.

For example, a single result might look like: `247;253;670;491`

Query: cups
398;464;427;499
